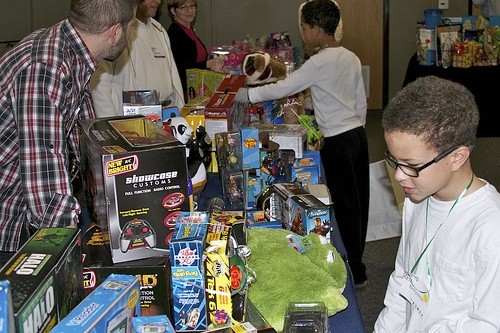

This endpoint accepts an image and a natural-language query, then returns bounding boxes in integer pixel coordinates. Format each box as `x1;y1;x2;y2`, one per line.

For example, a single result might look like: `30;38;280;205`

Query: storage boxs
416;15;500;66
0;67;333;333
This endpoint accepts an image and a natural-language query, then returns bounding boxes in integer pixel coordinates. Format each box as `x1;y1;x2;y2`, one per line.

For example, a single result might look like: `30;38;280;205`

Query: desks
198;158;364;333
401;51;500;138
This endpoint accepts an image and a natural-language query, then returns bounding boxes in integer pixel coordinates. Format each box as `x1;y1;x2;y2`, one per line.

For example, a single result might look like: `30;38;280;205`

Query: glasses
177;4;196;10
383;144;472;177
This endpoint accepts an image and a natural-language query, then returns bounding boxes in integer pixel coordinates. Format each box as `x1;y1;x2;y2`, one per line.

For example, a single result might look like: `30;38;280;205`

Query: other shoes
353;271;367;288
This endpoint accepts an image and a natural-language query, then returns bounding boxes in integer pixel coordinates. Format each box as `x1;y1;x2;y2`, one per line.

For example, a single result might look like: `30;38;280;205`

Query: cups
424;9;442;29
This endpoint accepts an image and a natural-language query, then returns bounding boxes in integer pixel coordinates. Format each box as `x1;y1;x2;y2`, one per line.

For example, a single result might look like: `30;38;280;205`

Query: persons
372;76;500;333
0;0;138;270
167;0;220;105
233;0;370;288
89;0;185;118
